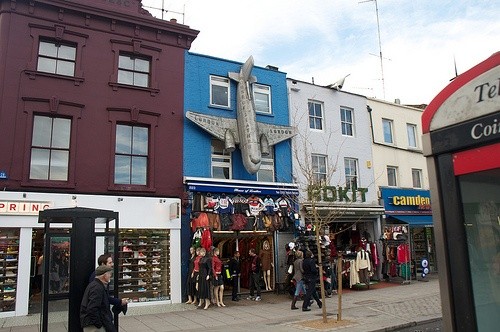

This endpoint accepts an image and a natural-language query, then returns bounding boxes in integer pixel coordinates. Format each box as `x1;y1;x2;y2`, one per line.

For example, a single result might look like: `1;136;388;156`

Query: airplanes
185;55;298;174
